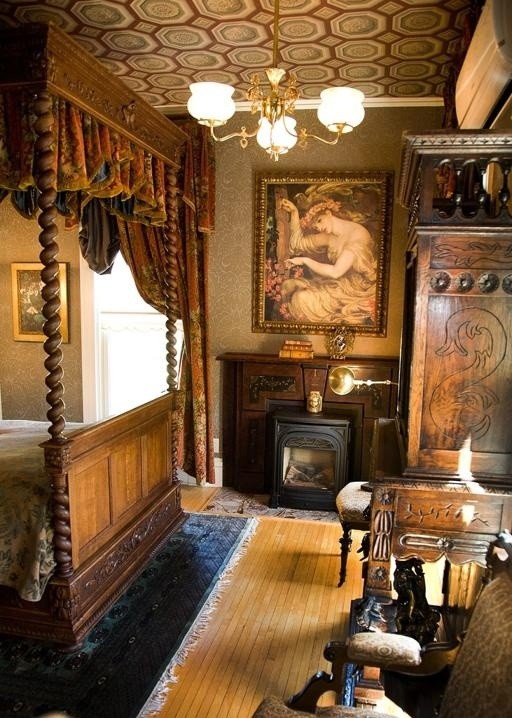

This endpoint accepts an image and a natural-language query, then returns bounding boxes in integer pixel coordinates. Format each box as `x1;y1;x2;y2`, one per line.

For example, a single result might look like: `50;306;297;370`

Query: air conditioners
454;2;512;130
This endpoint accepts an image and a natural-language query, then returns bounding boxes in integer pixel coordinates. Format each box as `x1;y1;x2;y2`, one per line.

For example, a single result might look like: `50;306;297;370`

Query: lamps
183;0;366;160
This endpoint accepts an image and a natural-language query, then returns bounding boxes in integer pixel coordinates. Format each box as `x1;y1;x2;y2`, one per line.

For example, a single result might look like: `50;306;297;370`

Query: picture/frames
251;169;394;338
10;260;71;345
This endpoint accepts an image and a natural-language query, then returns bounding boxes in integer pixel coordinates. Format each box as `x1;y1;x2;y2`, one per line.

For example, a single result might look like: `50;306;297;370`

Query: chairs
257;530;512;718
336;478;374;588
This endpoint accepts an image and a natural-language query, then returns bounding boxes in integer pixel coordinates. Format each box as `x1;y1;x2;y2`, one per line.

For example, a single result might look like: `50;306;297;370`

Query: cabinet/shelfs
396;131;511;485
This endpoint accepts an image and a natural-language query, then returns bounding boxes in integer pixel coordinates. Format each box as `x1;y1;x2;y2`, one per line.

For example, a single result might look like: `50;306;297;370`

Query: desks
364;416;511;628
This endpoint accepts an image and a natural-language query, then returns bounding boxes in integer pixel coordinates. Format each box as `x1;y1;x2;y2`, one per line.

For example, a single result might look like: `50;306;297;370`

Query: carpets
0;511;255;718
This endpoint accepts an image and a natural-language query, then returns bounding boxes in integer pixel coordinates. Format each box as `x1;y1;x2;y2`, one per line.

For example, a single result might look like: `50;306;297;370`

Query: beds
1;388;188;648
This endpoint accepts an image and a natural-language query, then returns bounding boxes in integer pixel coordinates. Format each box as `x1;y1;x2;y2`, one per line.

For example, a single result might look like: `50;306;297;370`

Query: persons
278;198;377;324
